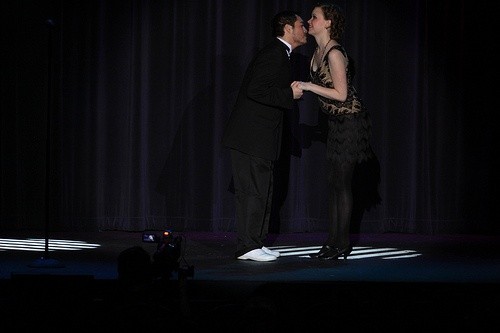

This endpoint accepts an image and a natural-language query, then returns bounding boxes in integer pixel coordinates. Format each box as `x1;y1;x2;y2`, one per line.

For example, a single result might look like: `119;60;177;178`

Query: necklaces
310;39;331;79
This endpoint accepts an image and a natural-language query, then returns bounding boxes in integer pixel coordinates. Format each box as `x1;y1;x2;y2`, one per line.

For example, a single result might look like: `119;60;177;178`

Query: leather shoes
237;249;276;262
262;247;280;257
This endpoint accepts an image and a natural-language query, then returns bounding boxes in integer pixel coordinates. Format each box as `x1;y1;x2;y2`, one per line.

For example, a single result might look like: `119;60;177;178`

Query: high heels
310;244;340;261
317;245;352;262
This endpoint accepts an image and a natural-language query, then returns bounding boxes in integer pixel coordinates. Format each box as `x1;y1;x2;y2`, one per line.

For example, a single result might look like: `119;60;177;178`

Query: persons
302;0;374;259
221;10;308;260
